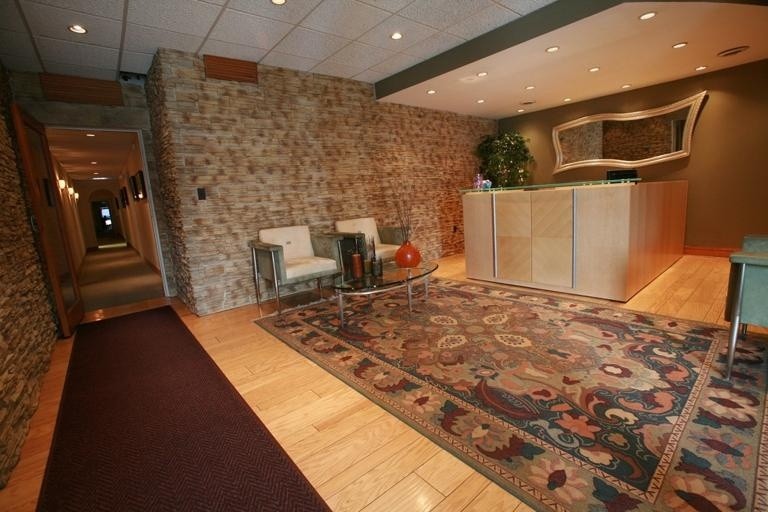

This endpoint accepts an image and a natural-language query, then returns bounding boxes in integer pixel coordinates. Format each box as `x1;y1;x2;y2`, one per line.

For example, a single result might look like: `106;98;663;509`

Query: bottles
350;235;383;278
474;173;491;192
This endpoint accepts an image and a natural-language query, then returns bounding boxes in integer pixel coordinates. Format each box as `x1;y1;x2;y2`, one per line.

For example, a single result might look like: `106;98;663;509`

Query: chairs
721;234;768;380
248;225;337;318
335;217;407;283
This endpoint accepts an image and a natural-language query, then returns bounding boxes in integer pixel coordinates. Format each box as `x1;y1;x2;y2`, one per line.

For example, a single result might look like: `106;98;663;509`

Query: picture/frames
129;170;147;201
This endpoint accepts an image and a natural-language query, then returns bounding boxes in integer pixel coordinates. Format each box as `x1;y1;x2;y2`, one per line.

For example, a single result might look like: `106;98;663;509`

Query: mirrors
552;90;708;176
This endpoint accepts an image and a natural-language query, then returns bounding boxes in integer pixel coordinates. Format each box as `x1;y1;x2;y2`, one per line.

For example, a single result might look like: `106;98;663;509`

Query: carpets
35;305;330;512
254;275;768;511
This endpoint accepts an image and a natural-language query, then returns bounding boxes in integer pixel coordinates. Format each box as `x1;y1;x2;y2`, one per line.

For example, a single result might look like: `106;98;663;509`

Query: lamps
58;174;66;189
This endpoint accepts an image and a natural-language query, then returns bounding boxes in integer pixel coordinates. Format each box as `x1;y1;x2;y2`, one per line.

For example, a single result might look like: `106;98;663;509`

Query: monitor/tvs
115;170;147;210
607;170;637;185
